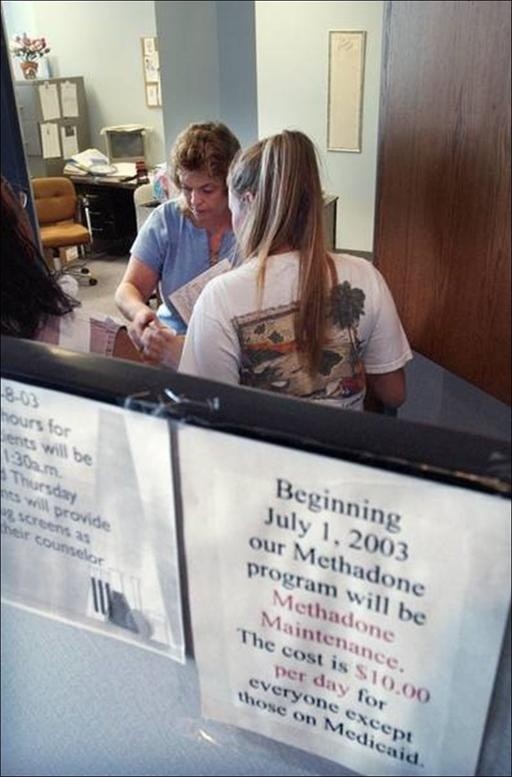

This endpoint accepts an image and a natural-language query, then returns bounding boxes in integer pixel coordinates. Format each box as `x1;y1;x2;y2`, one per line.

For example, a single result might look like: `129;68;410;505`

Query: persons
113;117;246;352
1;179;83;364
141;130;417;414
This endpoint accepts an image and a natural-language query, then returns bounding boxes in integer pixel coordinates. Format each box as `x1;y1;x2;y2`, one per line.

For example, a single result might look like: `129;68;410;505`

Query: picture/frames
328;28;365;155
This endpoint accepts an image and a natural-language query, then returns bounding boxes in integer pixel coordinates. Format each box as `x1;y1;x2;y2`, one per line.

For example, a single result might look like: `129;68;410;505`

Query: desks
66;165;157;257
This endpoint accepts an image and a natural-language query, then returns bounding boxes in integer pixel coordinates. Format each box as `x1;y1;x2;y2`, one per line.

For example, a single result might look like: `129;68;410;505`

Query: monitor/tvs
105;128;148;163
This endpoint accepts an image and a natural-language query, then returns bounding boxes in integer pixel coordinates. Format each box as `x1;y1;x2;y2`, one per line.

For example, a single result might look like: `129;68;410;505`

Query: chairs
102;124;148;160
29;177;100;285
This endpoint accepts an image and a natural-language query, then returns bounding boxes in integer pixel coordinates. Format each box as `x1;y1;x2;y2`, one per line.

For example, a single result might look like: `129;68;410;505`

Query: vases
21;59;38;78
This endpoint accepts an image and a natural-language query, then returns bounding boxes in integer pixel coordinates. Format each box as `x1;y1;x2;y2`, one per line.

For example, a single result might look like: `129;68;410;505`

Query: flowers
10;34;49;59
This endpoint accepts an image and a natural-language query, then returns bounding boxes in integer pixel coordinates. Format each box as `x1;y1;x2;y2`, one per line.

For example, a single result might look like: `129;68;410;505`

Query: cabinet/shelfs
15;76;91;175
